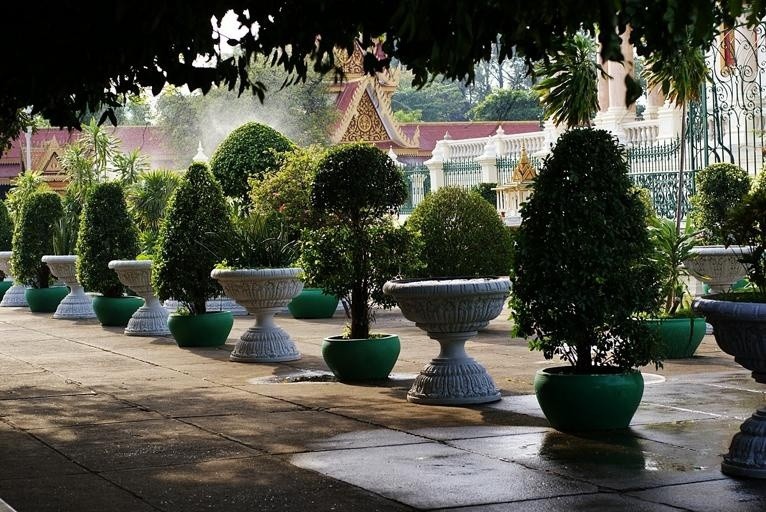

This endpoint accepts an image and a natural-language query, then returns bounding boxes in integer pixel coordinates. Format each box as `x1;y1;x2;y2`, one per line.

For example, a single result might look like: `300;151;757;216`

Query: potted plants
8;190;71;313
150;160;236;347
109;250;170;336
41;214;101;319
195;211;306;364
508;128;650;438
245;141;339;320
630;213;706;360
0;170;48;307
681;163;766;482
306;142;412;384
75;182;147;327
